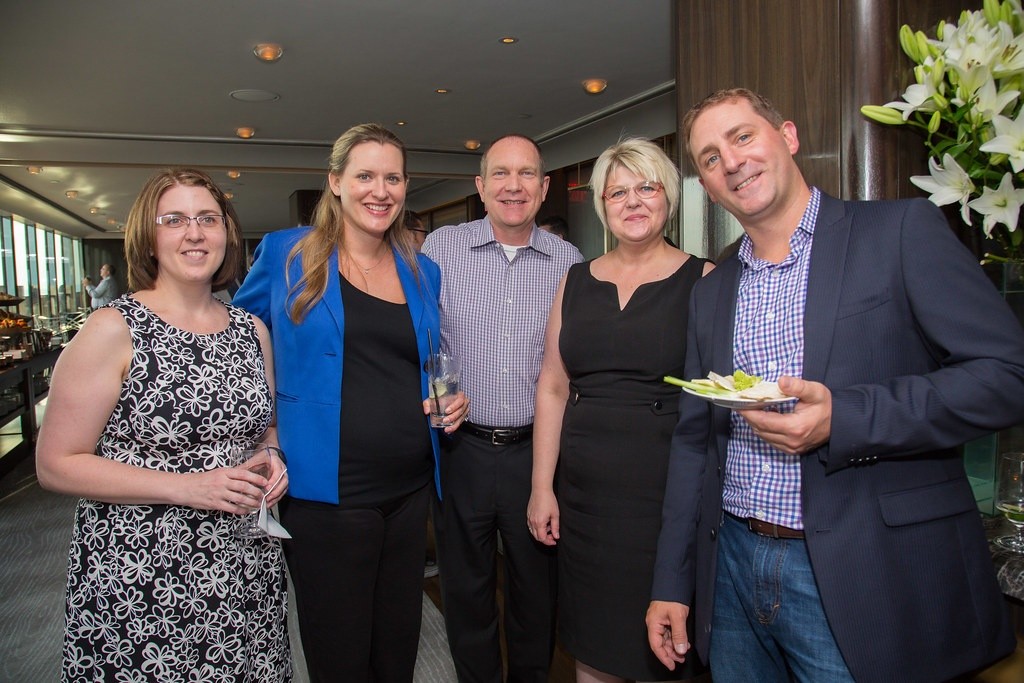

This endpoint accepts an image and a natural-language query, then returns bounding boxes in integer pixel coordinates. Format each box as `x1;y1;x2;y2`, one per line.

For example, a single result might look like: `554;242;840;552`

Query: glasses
155;214;226;233
602;180;663;203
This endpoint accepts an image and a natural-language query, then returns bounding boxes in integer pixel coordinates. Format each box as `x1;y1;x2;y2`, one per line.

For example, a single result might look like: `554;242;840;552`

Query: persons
230;124;470;683
36;166;294;683
645;89;1024;683
403;209;428;253
526;134;716;683
539;216;571;243
420;134;586;683
83;264;119;310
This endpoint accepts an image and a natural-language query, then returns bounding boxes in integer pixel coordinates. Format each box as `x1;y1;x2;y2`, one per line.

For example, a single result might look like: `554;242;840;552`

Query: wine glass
992;452;1024;552
230;443;272;539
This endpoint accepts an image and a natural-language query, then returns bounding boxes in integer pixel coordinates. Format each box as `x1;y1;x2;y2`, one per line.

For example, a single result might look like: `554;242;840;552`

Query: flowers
860;0;1024;266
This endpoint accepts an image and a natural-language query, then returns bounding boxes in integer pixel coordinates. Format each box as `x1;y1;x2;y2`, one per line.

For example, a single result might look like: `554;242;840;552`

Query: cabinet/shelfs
0;343;67;479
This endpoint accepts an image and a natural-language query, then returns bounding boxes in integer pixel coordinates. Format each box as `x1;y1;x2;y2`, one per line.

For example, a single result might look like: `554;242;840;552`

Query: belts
724;512;806;539
461;423;534;447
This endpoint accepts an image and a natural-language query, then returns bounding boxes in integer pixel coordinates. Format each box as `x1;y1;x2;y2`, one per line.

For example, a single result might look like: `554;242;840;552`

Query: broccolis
732;370;761;390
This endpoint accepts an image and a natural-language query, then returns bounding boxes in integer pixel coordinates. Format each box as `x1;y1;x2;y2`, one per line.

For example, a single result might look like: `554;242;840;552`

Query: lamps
27;165;41;174
583;78;607;93
253;44;283;60
236;127;255;138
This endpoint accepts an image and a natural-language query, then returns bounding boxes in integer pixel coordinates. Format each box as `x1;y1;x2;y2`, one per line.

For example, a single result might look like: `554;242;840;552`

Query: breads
0;318;26;329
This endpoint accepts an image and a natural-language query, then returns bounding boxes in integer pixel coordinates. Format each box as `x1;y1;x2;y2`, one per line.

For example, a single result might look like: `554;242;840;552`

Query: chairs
33;307;92;336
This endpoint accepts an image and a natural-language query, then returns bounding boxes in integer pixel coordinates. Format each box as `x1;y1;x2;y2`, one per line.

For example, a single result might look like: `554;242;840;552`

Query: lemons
430;383;448;399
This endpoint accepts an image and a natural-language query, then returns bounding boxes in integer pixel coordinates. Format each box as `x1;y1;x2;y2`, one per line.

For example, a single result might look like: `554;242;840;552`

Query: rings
529;527;533;530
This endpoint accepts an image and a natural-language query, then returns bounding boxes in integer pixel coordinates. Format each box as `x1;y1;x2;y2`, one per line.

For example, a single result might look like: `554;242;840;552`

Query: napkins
258;469;292;539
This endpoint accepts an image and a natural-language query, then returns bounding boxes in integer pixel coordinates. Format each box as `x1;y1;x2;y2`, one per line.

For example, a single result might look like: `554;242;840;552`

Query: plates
683;380;797;411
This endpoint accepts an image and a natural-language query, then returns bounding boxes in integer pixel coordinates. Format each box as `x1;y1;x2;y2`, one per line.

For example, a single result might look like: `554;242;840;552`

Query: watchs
260;447;287;465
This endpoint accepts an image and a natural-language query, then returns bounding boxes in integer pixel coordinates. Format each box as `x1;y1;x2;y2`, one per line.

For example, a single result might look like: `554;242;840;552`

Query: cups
429;353;464;427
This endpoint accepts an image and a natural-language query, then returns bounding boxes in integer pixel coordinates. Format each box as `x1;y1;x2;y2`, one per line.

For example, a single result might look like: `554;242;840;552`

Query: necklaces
341;240;388;273
